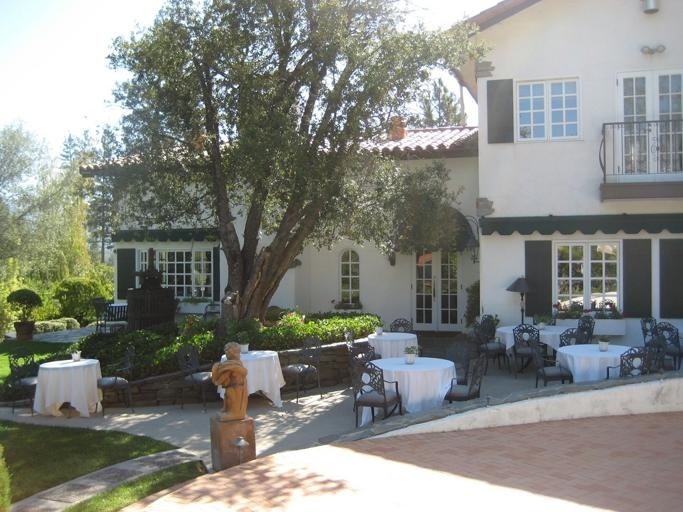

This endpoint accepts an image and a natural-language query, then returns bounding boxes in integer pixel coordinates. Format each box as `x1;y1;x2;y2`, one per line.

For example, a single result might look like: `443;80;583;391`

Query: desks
107;302;128;318
214;350;286;408
32;357;103;418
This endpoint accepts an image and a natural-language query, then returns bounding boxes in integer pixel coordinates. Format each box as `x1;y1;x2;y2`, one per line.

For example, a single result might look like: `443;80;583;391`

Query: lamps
462;235;479;265
639;44;665;56
504;277;536;323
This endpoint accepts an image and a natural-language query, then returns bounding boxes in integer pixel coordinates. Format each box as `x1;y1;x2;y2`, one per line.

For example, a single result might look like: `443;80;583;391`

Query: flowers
552;299;620;313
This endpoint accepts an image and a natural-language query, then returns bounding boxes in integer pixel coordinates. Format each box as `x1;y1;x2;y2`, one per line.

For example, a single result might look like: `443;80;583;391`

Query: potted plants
5;287;42;342
66;336;86;361
235;330;250;353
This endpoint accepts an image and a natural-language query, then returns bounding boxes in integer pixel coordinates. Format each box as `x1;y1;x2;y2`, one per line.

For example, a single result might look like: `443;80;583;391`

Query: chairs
8;350;39;416
281;336;322;404
202;303;220;318
91;296;127;334
93;343;137;416
174;343;220;414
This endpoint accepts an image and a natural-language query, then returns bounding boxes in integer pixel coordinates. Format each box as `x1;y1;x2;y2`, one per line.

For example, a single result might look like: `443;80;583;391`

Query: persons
210;340;249;423
